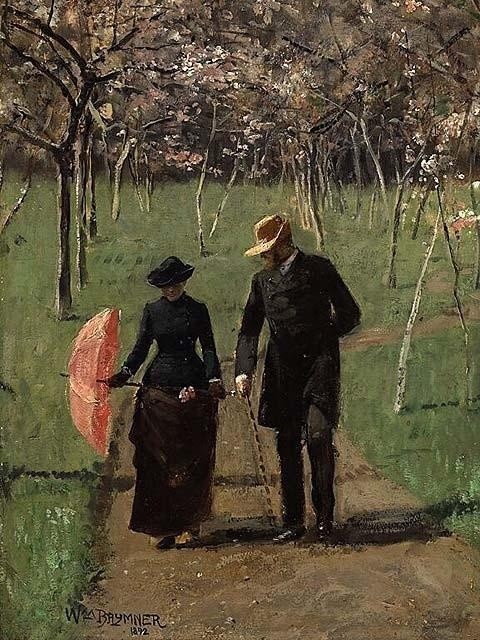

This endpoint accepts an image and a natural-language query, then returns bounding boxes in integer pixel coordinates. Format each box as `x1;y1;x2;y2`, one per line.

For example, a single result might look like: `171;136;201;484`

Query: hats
242;214;291;258
146;253;195;288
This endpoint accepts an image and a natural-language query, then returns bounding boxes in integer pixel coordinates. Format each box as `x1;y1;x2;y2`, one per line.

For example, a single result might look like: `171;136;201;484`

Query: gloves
104;368;131;388
209;379;226;399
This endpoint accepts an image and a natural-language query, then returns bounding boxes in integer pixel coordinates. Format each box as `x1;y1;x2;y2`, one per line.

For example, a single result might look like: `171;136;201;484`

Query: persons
230;211;364;548
102;255;229;550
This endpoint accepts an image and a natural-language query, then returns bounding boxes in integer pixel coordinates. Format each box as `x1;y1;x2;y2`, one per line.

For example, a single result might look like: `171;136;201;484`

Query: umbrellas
56;304;145;460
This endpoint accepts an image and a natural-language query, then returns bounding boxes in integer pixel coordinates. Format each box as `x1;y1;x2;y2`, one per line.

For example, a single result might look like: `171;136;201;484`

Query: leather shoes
273;524;307;543
315;520;334;542
155;536;177;549
